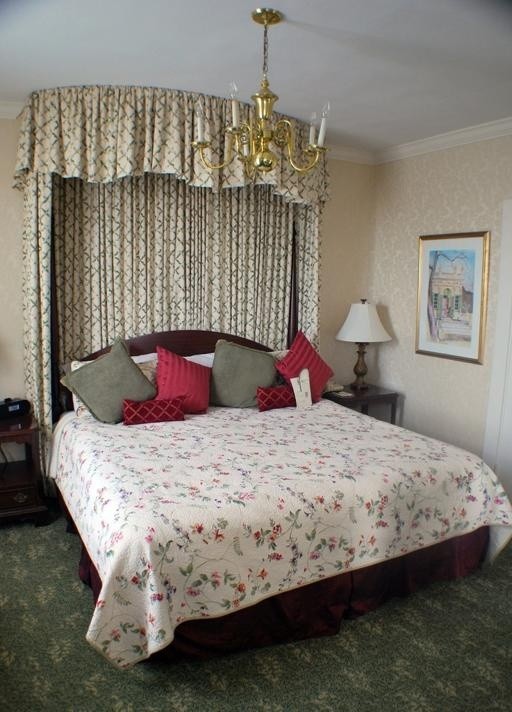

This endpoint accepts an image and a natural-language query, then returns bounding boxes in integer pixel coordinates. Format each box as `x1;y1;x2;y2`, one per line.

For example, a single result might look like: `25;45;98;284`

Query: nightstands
0;415;50;527
324;383;398;425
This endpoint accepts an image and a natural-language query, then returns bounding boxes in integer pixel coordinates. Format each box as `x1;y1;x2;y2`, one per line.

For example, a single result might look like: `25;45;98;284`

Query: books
332;390;353;398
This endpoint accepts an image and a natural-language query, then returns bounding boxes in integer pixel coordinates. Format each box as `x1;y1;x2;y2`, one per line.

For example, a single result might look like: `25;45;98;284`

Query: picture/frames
414;230;490;365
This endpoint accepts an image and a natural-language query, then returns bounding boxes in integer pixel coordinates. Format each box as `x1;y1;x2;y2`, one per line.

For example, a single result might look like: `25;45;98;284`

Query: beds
46;328;512;672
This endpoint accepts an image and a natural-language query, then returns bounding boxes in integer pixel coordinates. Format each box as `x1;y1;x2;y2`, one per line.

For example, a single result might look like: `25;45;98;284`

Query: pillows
58;335;334;425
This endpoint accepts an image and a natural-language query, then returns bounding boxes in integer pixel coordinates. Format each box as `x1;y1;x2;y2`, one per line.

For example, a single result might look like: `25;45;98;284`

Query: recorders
0;398;31;420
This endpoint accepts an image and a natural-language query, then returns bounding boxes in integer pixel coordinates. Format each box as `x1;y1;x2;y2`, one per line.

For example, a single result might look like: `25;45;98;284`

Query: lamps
334;298;392;391
190;9;331;178
